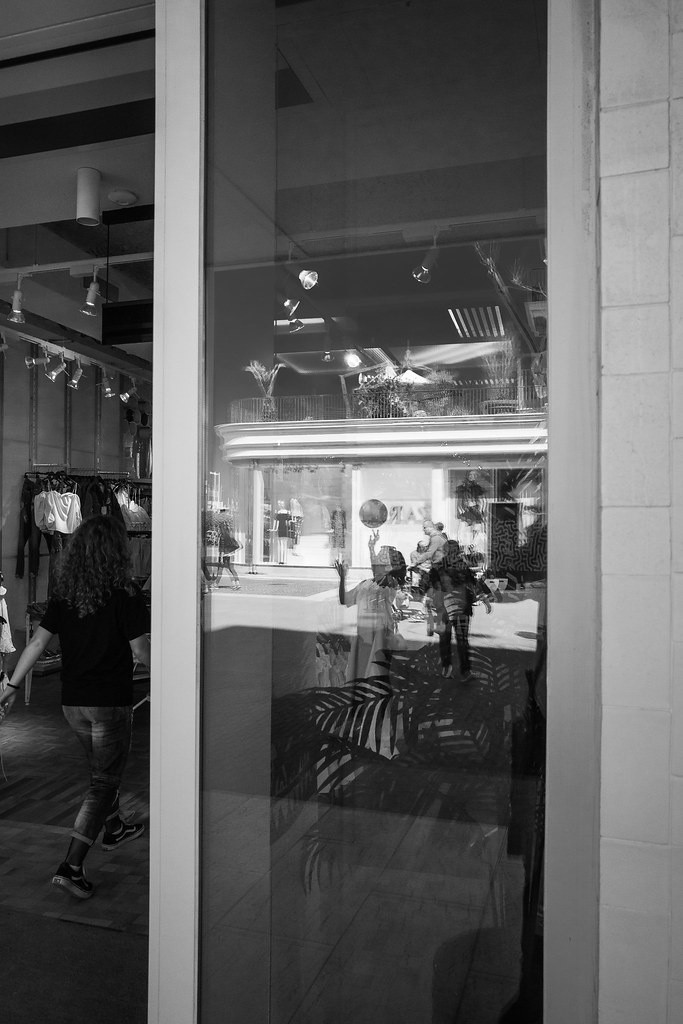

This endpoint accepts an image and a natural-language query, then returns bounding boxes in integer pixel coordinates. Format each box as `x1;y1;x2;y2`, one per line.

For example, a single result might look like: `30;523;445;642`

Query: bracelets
7;683;20;690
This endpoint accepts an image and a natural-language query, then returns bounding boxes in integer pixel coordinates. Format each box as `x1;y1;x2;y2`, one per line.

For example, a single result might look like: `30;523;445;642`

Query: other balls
360;499;388;527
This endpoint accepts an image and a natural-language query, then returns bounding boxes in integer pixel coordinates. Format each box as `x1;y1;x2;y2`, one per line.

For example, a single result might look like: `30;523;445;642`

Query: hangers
114;472;136;494
36;463;80;489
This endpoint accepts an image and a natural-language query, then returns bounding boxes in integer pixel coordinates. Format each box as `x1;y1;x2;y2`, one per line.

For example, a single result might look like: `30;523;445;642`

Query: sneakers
102;824;145;851
52;863;95;898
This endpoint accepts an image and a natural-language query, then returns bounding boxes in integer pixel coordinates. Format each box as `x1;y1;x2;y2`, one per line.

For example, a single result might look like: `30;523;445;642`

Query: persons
210;509;492;735
0;514;151;898
273;500;291;565
327;504;348;565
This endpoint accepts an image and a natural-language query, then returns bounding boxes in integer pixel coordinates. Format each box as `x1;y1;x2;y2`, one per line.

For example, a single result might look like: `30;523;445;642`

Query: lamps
7;274;26;323
45;352;66;382
25;348;50;368
67;357;84;390
120;376;136;403
102;367;115;397
79;266;99;316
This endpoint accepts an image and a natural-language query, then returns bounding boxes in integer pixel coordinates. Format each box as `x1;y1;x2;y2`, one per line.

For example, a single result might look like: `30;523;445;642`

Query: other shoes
462;671;471;681
36;652;54;663
27;603;45;615
46;650;60;661
442;666;452;677
214;585;219;589
233;583;241;590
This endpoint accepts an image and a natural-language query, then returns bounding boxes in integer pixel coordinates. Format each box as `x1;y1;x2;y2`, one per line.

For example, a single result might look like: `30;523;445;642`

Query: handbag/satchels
387;634;407;656
225;538;239;555
